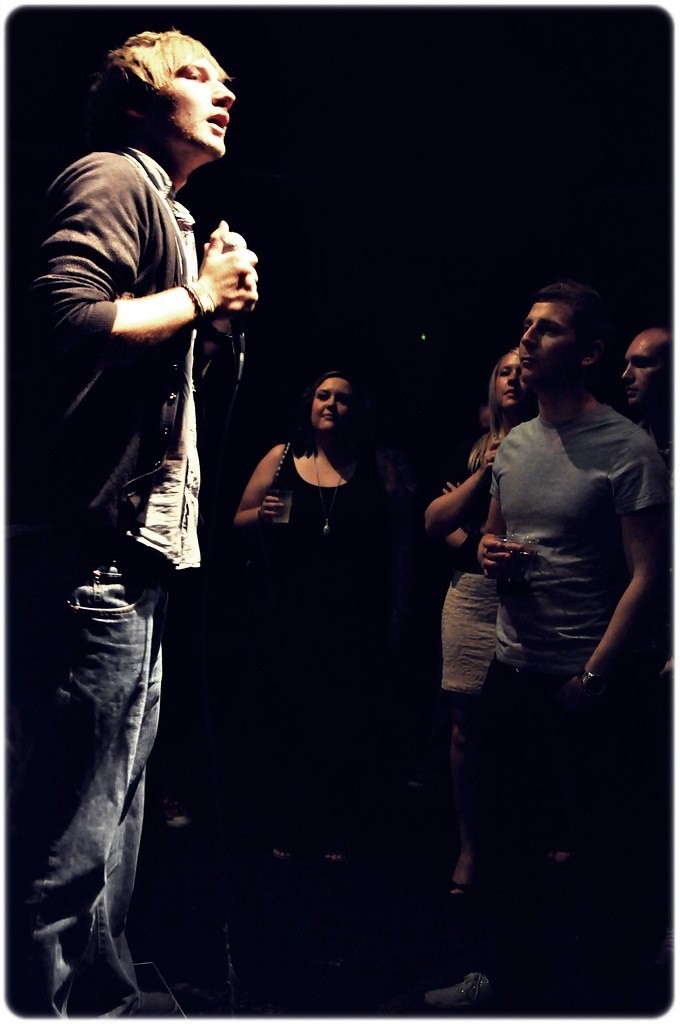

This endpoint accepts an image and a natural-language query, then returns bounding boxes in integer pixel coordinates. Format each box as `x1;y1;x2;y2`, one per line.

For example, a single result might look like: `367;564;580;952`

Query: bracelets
180;283;205;322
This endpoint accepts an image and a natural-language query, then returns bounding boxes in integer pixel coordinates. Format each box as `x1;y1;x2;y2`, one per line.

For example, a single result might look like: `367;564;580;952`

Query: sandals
444;870;474;895
324;833;349;860
272;835;297;858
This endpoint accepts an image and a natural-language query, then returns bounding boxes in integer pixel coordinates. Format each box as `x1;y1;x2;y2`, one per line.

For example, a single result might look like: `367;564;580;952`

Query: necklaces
313;448;348;536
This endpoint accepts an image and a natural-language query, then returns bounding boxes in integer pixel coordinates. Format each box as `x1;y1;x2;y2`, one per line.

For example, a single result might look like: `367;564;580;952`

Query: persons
6;26;259;1019
232;279;673;1018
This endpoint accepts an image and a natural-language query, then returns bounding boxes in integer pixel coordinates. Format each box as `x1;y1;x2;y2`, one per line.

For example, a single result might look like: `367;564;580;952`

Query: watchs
577;668;610;697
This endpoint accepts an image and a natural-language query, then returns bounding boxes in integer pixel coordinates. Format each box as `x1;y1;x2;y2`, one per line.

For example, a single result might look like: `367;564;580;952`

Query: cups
269;490;292;524
494;534;539;596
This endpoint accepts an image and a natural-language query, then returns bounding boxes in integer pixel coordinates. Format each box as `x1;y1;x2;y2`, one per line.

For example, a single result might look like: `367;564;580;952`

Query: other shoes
158;793;192;829
424;969;512;1007
136;990;177;1015
